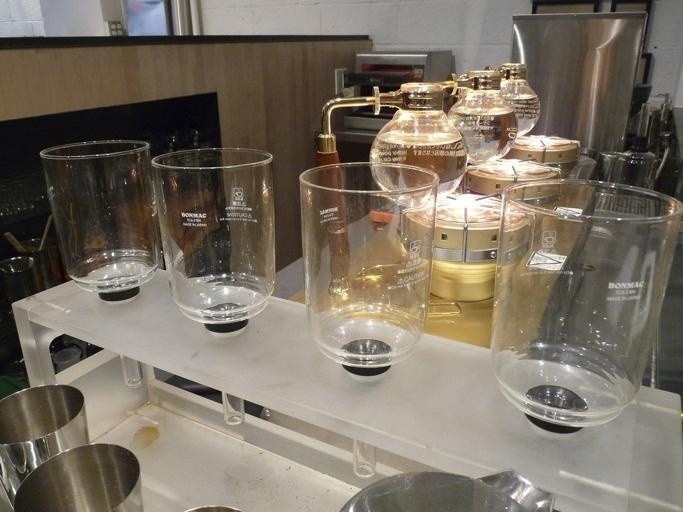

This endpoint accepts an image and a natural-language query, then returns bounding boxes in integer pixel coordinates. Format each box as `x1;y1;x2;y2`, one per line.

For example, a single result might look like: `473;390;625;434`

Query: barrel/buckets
1;385;91;510
186;506;242;511
339;470;529;512
15;443;147;511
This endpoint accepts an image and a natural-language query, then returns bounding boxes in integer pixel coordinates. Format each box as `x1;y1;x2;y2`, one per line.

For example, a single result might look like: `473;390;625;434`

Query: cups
290;161;435;369
35;138;161;297
150;143;277;330
485;177;683;430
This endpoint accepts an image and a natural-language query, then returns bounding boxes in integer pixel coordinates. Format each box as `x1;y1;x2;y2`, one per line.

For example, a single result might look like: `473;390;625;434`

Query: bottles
369;61;541;208
